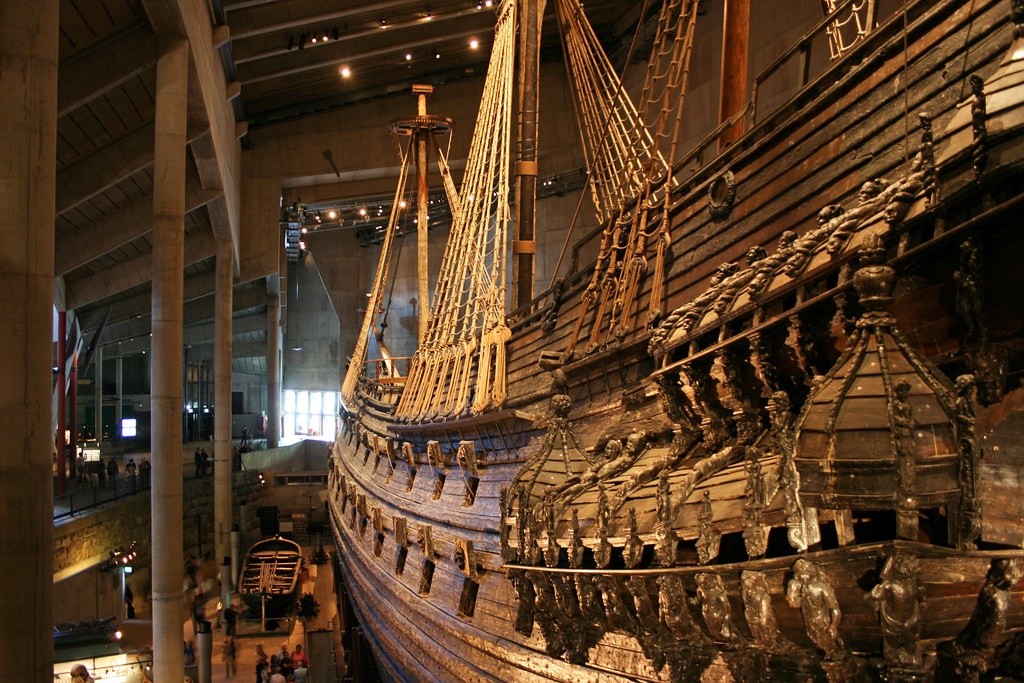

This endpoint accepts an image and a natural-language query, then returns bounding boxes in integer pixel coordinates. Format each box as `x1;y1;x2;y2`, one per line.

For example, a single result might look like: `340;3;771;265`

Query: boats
325;0;1024;683
238;532;302;614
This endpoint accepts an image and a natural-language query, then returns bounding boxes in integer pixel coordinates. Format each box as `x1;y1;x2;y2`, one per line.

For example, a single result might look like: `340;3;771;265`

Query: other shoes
224;640;227;643
231;640;234;642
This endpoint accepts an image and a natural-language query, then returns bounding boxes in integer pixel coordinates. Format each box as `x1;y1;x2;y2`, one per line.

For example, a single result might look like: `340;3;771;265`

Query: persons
223;636;237;677
124;582;136;620
194;447;208;478
77;452;87;476
184;640;195;666
224;604;240;636
71;664;94;683
239;444;253;453
256;645;308;683
125;458;151;488
192;595;206;634
97;457;119;487
240;425;247;447
